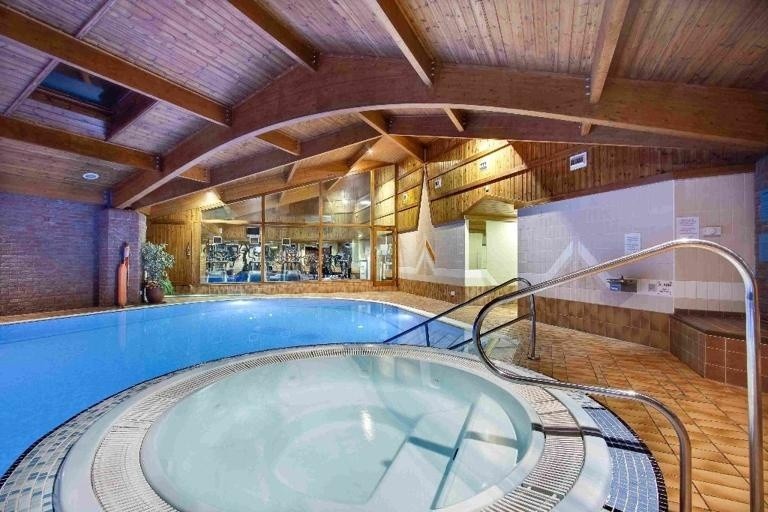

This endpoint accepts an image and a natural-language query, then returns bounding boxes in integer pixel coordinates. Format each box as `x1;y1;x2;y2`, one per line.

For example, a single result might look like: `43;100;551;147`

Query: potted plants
141;241;175;304
